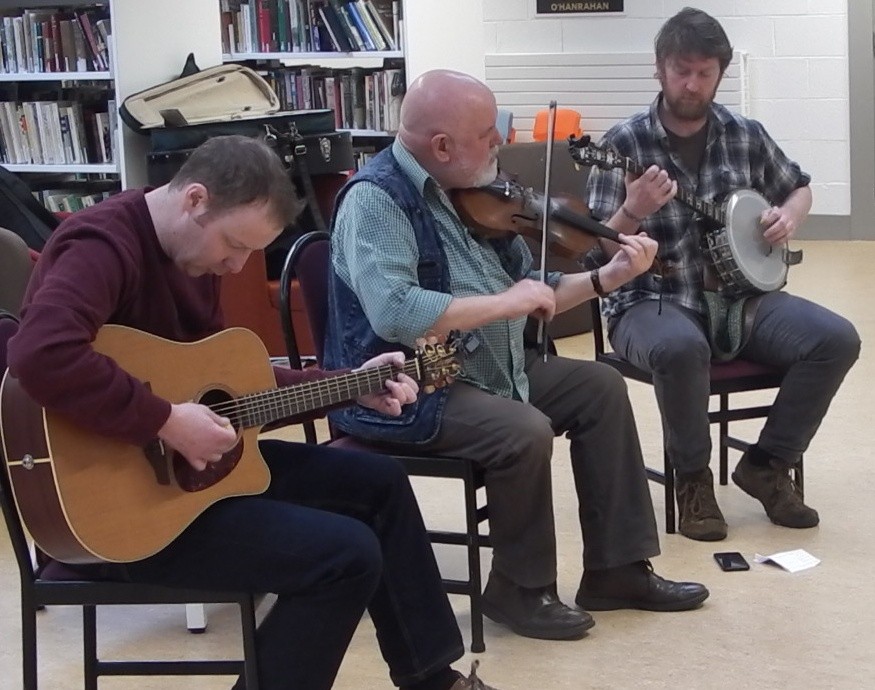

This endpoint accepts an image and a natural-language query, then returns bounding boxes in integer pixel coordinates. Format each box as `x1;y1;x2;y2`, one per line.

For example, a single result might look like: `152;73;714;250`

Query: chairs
531;106;584;144
492;107;517;146
580;239;808;536
0;227;261;690
276;230;501;659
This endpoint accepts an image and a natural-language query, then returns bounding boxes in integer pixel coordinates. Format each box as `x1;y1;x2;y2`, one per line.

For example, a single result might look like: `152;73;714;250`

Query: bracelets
621;204;648;223
590;268;609;298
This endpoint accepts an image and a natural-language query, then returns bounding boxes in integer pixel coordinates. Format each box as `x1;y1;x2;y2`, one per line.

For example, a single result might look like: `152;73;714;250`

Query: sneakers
732;447;820;528
450;658;499;690
674;465;728;542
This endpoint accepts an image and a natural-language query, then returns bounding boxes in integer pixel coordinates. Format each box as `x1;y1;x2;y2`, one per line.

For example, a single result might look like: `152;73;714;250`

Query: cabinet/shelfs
0;0;128;217
218;0;411;184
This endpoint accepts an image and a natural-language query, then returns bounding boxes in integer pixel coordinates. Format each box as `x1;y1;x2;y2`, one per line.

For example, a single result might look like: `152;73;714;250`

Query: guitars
0;322;469;565
568;132;803;298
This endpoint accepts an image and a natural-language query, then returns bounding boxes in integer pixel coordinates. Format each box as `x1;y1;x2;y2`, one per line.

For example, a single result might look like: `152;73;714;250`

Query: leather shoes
575;559;710;611
481;569;596;639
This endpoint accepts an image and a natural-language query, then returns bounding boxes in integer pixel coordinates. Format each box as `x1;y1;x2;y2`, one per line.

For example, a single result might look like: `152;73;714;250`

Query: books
1;7;122;214
219;0;407;133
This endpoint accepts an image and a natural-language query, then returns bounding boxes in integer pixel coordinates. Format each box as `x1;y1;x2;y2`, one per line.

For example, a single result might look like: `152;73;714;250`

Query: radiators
482;49;751;158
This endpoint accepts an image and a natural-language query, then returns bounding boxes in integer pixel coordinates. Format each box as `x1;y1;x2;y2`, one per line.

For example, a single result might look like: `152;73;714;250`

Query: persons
583;7;861;541
323;69;710;639
6;136;496;690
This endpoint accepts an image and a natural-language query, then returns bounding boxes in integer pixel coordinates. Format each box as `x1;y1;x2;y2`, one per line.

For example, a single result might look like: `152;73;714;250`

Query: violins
450;167;667;274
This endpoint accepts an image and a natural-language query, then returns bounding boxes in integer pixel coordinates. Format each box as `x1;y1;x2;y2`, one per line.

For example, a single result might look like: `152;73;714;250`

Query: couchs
210;244;319;366
